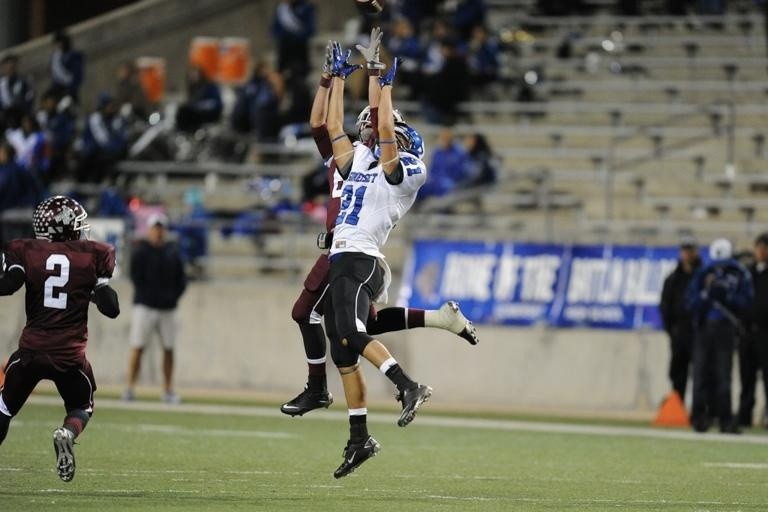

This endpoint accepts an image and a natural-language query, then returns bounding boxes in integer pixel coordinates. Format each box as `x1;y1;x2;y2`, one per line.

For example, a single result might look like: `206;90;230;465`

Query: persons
0;26;84;146
267;1;499;128
118;211;187;404
730;232;768;427
281;27;478;417
1;55;280;213
660;240;703;402
330;42;431;479
169;185;210;263
412;127;490;207
0;196;121;481
683;238;753;435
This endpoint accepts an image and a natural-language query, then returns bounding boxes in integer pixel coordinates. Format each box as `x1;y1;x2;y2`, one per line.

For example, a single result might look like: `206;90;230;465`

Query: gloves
357;26;383;70
332;42;363;78
322;38;343;79
377;56;398;88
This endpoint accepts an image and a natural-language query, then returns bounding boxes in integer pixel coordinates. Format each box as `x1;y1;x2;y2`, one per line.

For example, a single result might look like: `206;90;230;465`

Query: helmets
355;109;405;146
33;195;91;244
373;119;424;161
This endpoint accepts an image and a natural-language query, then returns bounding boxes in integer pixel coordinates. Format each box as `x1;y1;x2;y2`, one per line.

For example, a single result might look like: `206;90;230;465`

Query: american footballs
356;1;386;19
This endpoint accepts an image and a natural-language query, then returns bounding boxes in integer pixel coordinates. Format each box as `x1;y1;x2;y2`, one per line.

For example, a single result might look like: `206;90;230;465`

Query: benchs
0;12;768;279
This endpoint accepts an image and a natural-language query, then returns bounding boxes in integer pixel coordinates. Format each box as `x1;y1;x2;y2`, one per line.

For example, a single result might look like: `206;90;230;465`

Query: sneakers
439;299;479;345
393;383;433;427
334;435;380;478
51;424;75;483
280;384;334;416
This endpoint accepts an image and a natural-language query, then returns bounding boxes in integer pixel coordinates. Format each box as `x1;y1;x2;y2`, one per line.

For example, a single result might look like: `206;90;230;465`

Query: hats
146;211;168;229
